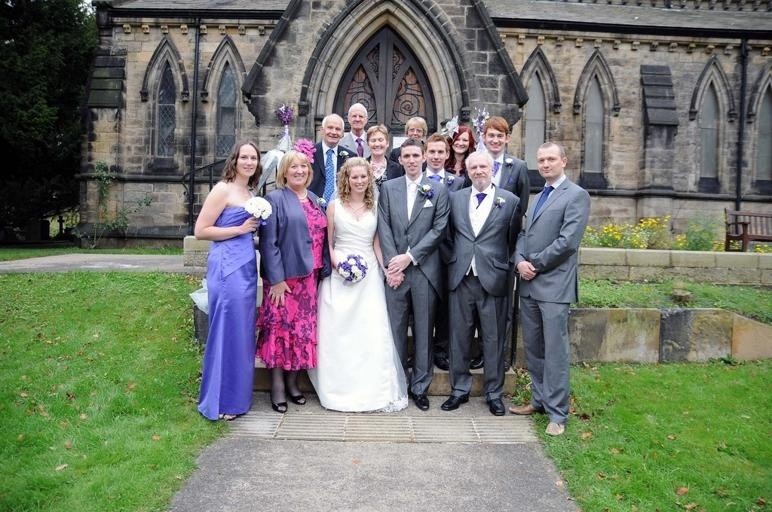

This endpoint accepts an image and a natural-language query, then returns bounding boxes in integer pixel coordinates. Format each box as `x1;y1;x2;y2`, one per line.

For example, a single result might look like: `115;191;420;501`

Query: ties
356;137;366;160
430;174;442;185
491;160;501;178
475;193;487;209
533;185;555;226
323;149;336;207
406;182;415;221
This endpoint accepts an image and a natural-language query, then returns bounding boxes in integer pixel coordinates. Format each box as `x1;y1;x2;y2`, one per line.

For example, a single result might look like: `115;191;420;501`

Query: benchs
724;208;772;252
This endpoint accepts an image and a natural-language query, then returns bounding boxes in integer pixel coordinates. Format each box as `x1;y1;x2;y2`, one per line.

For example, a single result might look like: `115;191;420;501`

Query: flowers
245;196;272;233
336;255;367;283
316;149;514;208
472;107;491;152
273;104;294;152
293;139;316;163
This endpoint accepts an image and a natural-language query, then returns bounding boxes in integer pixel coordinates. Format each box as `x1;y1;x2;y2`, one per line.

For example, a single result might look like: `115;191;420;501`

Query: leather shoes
440;392;471;411
408;387;430;412
544;421;565;438
486;397;506;417
502;360;511;370
433;354;451;372
508;400;540;418
469;355;486;370
404;355;416;369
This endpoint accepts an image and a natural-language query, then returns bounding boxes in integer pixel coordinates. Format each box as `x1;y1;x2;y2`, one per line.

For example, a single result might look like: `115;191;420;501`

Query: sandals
270;401;287;414
288;392;306;405
